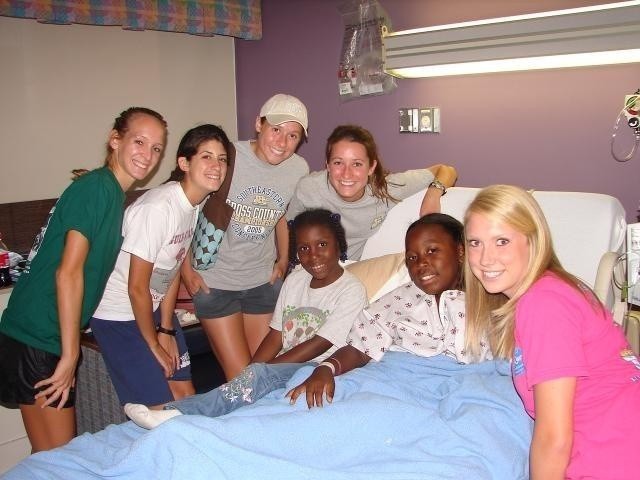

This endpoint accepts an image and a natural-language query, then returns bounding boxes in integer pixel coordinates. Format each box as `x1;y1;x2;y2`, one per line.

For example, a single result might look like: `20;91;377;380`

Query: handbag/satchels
191;193;235;270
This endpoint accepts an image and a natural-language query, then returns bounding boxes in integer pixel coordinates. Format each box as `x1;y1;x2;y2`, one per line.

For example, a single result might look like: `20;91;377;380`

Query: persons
90;123;228;412
124;208;366;431
0;106;168;456
284;213;493;410
464;184;640;480
180;94;310;384
284;125;458;261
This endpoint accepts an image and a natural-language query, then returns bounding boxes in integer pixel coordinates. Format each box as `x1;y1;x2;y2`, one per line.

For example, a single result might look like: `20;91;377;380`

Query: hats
260;93;309;138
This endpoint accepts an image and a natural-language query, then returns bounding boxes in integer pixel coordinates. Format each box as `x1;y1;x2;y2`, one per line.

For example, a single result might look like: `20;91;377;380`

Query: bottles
0;233;10;290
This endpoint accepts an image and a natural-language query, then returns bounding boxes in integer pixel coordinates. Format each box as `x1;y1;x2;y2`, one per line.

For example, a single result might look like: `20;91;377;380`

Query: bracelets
315;361;335;376
331;357;341;376
427;181;447;196
156;323;177;336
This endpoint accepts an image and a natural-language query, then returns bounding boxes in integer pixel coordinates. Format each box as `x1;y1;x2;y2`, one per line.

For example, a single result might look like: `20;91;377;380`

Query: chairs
0;191;150;318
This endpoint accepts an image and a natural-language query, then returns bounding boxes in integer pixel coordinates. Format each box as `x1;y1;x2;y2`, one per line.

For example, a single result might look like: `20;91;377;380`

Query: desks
74;325;214;435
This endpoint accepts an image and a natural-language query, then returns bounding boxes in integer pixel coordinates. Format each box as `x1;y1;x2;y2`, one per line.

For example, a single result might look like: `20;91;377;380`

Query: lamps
380;1;640;79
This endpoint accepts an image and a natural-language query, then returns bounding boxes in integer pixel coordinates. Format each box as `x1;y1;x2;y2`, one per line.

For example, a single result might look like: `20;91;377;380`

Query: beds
1;188;629;480
